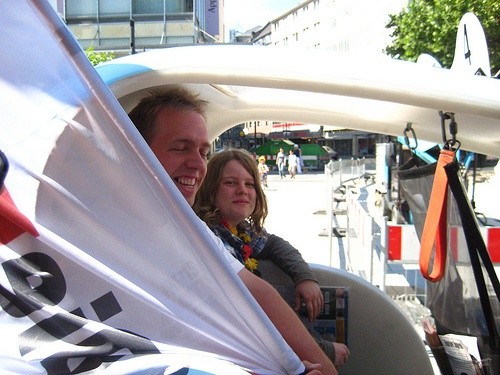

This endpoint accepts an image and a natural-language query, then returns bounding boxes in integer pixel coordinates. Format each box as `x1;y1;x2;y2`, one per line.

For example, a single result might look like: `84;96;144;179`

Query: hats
259;156;265;162
294;144;298;148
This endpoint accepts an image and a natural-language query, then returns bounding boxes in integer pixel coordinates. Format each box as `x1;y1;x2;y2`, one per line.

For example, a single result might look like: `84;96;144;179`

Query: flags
0;0;306;375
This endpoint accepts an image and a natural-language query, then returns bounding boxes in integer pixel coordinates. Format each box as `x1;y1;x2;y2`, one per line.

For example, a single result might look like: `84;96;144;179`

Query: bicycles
260;171;268;188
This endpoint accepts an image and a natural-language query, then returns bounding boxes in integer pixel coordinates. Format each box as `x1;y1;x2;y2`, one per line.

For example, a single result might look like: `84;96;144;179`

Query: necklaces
220;221;258;272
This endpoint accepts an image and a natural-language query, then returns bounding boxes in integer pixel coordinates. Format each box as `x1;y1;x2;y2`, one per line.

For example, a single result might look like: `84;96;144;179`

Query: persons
288;150;297;179
275;147;286;179
257;156;268;190
130;87;338;375
193;150;350;366
292;144;305;174
359;146;367;155
